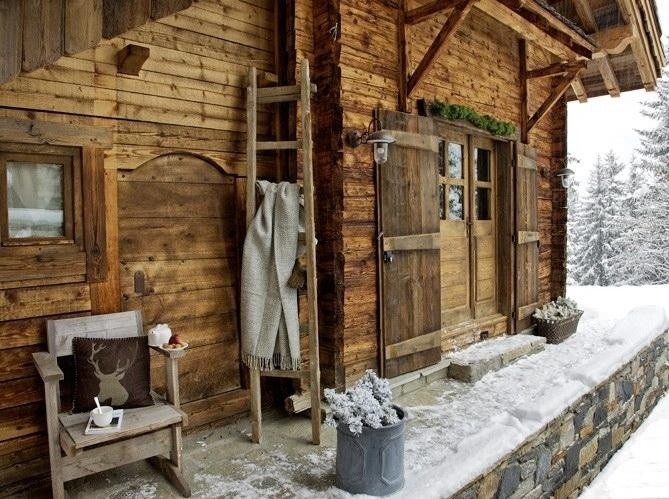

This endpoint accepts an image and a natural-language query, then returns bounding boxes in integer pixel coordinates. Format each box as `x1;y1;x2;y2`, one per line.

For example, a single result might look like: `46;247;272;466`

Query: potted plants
532;294;583;344
324;369;407;497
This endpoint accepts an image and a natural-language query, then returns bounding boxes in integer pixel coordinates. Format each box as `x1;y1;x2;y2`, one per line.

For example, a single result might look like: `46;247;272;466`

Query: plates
158;342;188;351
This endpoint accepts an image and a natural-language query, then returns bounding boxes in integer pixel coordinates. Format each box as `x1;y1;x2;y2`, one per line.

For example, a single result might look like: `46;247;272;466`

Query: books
84;408;125;434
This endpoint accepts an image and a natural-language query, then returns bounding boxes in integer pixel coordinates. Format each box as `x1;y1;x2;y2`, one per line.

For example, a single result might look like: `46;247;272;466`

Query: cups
90;406;114;427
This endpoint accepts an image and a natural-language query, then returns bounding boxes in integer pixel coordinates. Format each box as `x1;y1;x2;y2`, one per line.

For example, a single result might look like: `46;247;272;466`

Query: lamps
538;160;575;188
345;118;395;165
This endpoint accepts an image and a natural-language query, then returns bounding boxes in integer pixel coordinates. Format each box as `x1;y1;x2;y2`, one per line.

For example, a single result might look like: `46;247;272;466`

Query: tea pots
147;323;172;347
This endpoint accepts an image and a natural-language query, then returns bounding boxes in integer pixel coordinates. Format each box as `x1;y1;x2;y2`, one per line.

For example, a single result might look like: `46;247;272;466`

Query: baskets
529;308;585;345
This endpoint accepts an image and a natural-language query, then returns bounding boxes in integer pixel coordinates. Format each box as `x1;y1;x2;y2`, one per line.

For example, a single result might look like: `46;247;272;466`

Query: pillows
69;336;154;414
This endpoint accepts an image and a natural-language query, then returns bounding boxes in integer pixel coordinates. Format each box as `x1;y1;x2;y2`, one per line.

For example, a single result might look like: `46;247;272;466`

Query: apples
168;333;181;346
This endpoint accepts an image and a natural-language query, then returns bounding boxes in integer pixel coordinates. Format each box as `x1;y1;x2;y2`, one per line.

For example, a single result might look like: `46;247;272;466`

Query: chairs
31;309;191;499
